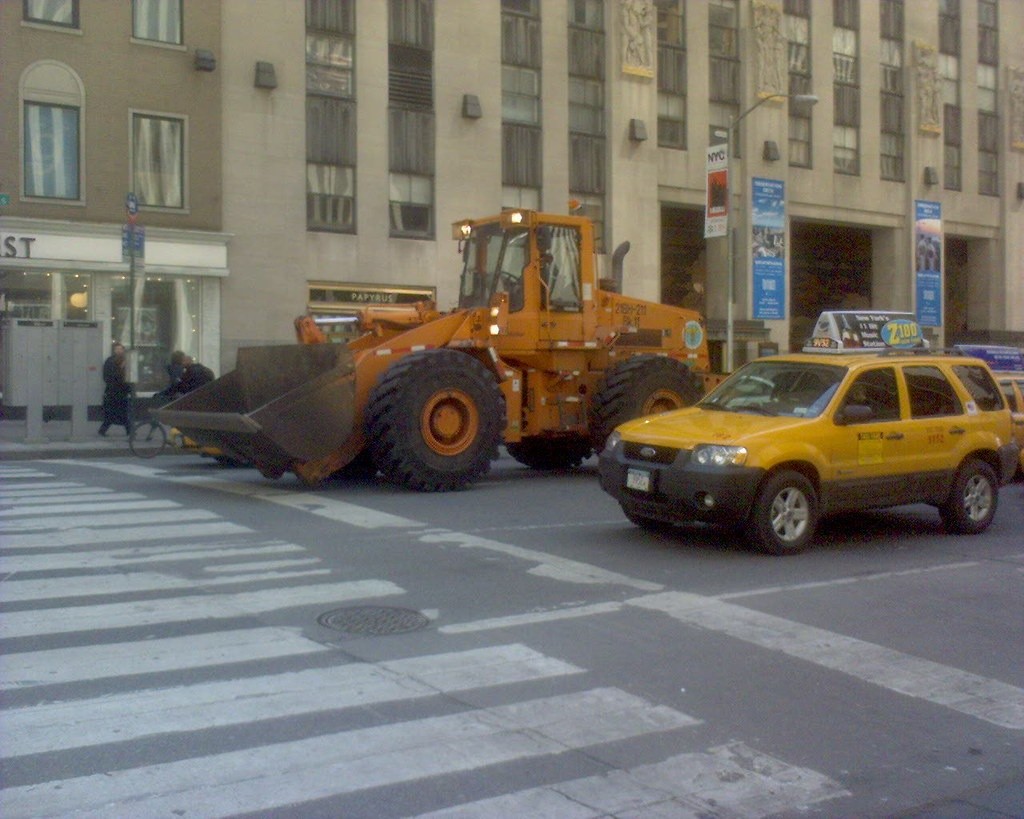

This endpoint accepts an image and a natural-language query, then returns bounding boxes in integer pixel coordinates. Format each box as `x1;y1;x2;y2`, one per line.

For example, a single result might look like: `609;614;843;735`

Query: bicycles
129;399;168;460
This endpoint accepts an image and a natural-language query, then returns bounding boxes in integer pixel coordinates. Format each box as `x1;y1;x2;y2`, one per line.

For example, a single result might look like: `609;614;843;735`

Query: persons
509;255;551;313
96;343;136;437
151;355;215;403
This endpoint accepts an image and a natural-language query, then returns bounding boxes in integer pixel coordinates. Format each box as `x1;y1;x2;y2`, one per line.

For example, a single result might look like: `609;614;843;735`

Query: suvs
597;307;1024;557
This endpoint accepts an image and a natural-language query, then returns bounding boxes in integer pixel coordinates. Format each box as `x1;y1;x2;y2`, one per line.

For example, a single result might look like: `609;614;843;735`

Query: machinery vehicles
150;204;735;493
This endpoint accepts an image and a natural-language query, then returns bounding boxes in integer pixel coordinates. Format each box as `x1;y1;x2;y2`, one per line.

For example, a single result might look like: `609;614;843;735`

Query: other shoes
127;431;136;436
98;430;107;437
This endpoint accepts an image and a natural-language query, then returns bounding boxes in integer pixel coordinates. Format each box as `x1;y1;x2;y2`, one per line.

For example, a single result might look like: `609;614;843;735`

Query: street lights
726;93;820;372
